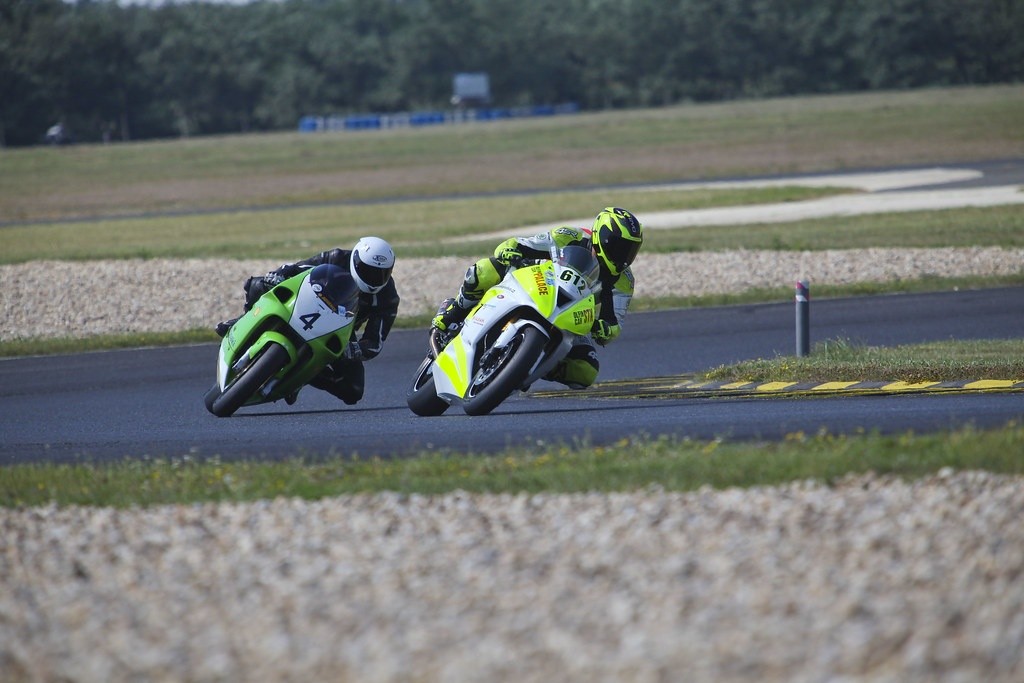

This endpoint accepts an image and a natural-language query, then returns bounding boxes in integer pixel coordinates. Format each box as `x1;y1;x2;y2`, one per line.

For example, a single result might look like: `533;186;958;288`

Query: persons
431;206;644;396
212;237;400;405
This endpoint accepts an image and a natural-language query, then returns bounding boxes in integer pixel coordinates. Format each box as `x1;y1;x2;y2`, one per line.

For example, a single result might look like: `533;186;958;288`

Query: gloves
342;343;358;361
265;265;292;285
591;318;613;349
500;247;522;268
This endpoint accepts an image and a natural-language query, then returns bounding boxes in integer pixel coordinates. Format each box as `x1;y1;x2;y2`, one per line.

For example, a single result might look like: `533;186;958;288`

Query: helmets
592;205;644;277
350;237;396;294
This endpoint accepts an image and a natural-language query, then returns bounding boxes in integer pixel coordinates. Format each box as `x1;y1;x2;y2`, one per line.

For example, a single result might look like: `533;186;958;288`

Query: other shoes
432;299;478;335
214;316;246;337
521;384;531;393
285;384;305;405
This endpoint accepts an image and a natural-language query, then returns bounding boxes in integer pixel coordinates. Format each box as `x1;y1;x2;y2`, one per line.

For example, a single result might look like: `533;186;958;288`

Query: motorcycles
405;245;607;417
201;263;380;417
